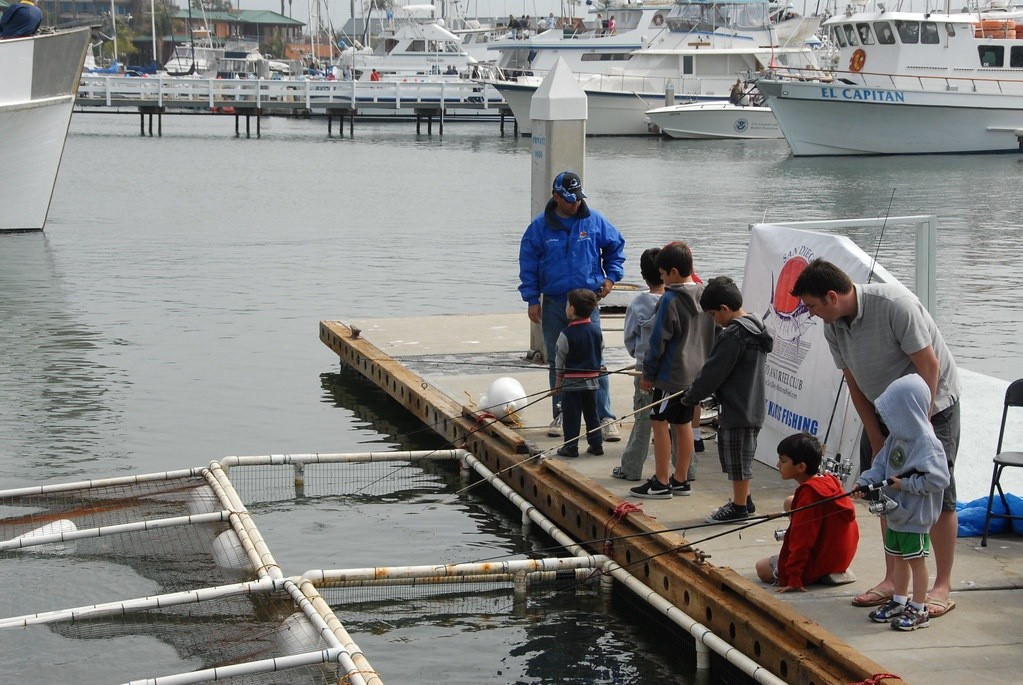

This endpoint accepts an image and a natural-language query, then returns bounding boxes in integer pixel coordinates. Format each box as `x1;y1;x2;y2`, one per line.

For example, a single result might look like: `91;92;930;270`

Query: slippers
852;589;911;607
924;597;956;618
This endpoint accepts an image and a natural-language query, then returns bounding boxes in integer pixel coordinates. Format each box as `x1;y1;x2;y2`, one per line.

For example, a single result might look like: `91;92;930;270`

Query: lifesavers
654;14;664;26
849;49;866;71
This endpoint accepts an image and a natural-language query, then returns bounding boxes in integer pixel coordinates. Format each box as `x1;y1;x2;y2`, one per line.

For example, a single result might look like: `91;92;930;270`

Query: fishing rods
390;364;637;439
821;188;897;483
497;461;954;612
353;390;558;493
436;511;787;569
350;358;642;377
421;387;718;511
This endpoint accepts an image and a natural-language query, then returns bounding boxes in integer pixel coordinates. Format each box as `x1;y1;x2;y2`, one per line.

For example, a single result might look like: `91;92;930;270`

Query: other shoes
822;568;855;585
587;445;603;455
557;445;579;457
613;467;635;480
694;438;704;454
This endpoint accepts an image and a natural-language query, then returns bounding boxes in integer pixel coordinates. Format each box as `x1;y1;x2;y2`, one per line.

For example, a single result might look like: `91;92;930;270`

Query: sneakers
548;412;564;436
629;474;673;499
712;503;756;516
704;497;749;523
891;604;930;630
600;417;621;441
868;598;911;623
670;473;691;496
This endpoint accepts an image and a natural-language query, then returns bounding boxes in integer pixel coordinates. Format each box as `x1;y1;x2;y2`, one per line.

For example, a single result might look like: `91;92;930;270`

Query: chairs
981;379;1022;546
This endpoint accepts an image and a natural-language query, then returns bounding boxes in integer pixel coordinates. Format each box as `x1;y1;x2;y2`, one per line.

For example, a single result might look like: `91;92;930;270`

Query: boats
1;23;105;235
108;1;1023;157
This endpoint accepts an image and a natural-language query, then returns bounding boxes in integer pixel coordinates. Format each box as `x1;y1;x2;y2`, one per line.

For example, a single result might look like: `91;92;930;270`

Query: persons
0;0;43;38
791;261;962;617
730;79;751;106
520;15;532;27
770;11;793;21
613;248;706;480
595;14;604;37
370;69;379;81
630;242;724;499
446;65;458;75
507;14;520;39
852;372;950;631
608;16;616;34
519;172;625;443
554;289;605;458
538;13;563;28
756;432;859;593
681;276;774;522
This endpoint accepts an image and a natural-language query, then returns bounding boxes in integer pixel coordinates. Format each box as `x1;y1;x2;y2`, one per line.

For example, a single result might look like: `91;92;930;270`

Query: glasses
560;196;581;203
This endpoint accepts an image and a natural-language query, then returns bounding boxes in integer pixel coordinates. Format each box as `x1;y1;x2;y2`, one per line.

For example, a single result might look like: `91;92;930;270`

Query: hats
553;171;588;203
